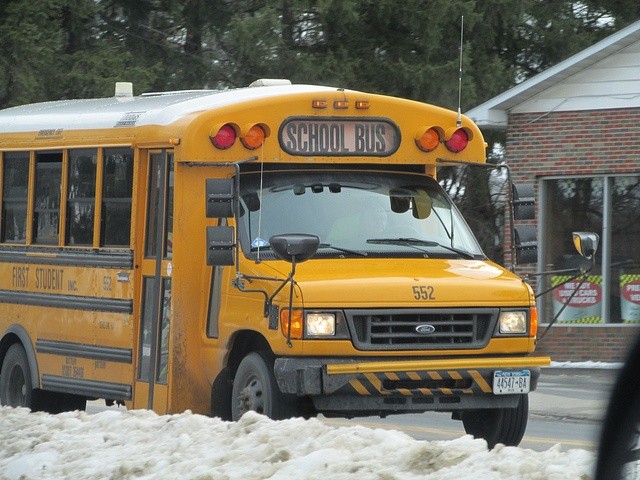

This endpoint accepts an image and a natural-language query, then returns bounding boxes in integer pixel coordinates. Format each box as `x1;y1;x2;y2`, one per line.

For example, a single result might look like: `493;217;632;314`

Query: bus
0;79;599;448
